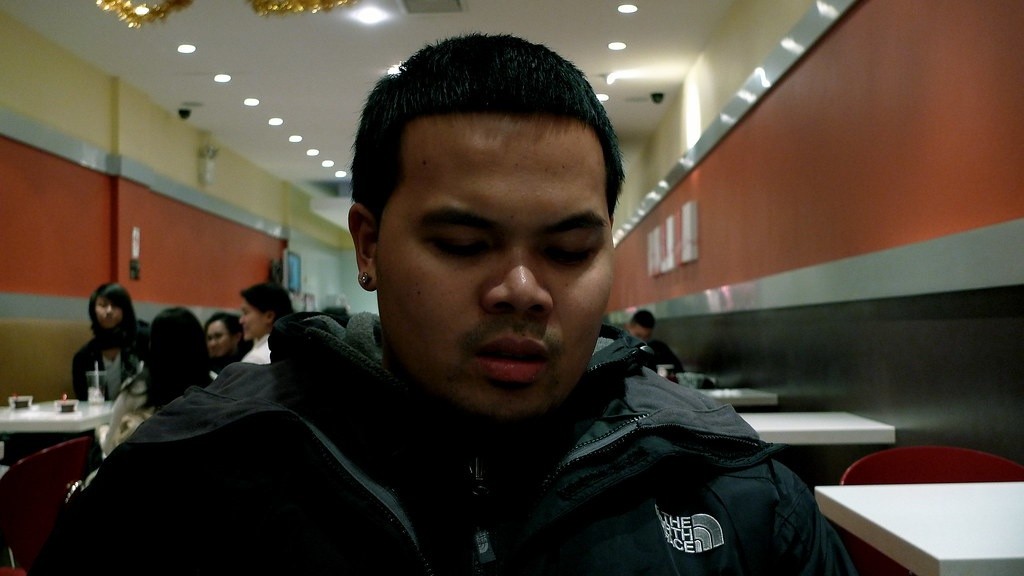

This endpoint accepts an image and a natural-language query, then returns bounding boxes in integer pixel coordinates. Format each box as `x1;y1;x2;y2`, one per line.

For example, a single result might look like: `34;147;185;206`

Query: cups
657;364;674;379
85;369;107;404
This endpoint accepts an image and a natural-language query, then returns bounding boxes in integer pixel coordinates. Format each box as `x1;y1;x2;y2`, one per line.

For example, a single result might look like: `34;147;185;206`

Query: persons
239;282;293;365
31;33;853;576
204;313;244;379
71;283;149;402
95;305;214;460
622;311;684;372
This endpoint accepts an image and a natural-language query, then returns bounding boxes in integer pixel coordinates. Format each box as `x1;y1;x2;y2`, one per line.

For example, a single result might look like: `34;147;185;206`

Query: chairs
839;445;1024;576
0;436;93;575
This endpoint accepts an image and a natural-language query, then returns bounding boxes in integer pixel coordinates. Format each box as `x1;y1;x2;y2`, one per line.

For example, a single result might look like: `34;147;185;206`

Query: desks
0;400;113;434
814;481;1023;576
699;388;779;406
740;411;895;445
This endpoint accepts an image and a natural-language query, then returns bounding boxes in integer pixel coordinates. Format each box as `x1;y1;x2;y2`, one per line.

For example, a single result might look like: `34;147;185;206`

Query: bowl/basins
7;395;33;410
54;399;79;413
